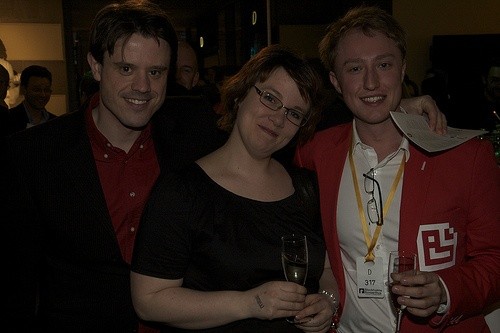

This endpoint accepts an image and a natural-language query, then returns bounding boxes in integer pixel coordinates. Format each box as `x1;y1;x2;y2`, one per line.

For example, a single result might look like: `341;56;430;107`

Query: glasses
363;167;383;225
252;84;310;128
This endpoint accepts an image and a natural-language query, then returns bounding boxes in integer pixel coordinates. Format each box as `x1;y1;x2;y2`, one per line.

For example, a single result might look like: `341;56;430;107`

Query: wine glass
282;234;309;325
389;251;416;333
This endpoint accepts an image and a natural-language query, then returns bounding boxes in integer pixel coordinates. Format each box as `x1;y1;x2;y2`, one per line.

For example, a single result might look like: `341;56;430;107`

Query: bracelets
318;289;339;317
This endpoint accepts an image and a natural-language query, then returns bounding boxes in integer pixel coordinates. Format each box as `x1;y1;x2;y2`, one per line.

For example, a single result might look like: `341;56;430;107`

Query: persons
290;7;500;333
130;43;341;333
0;63;100;141
0;0;448;333
174;41;200;91
454;62;500;132
207;65;229;85
